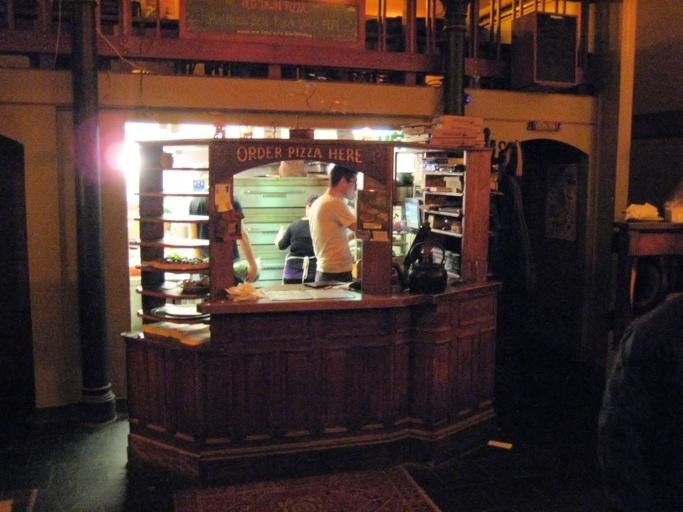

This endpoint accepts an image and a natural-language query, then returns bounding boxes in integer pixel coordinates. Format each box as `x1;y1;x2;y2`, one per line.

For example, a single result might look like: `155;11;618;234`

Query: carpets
171;462;443;511
0;489;40;511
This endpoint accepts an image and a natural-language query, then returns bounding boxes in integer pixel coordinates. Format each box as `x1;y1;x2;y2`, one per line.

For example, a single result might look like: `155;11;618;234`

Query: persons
190;195;257;282
274;166;357;284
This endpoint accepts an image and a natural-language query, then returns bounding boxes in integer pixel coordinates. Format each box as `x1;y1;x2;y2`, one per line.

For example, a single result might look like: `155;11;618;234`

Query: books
430;177;462;193
400;115;485;147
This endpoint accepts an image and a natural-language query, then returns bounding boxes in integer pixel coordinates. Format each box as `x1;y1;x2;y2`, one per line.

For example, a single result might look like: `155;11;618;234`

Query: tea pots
390;242;447;295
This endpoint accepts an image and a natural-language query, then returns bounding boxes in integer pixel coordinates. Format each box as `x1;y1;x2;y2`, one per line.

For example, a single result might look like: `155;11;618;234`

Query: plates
149;306;207;323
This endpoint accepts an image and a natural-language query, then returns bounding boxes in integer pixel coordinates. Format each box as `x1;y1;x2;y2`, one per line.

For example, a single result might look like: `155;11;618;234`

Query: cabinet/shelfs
423;151;490;281
128;140;213;346
